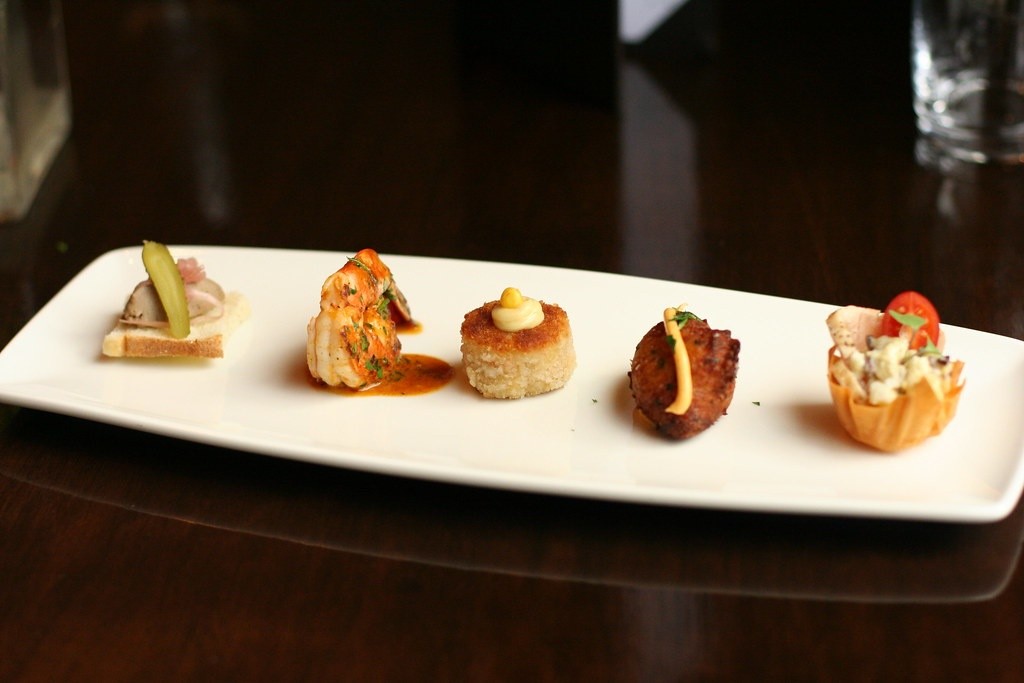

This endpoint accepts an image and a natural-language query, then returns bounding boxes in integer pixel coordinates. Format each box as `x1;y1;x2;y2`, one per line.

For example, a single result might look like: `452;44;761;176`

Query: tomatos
878;293;938;352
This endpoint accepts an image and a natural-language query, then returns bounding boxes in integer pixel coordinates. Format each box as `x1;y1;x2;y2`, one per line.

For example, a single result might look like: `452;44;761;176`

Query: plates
4;410;1022;605
1;244;1022;524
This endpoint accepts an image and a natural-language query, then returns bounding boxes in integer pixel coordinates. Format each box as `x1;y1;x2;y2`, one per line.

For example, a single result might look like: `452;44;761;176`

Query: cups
909;0;1024;168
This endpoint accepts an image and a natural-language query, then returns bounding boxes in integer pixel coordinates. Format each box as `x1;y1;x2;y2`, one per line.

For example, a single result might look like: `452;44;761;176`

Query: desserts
825;290;966;453
